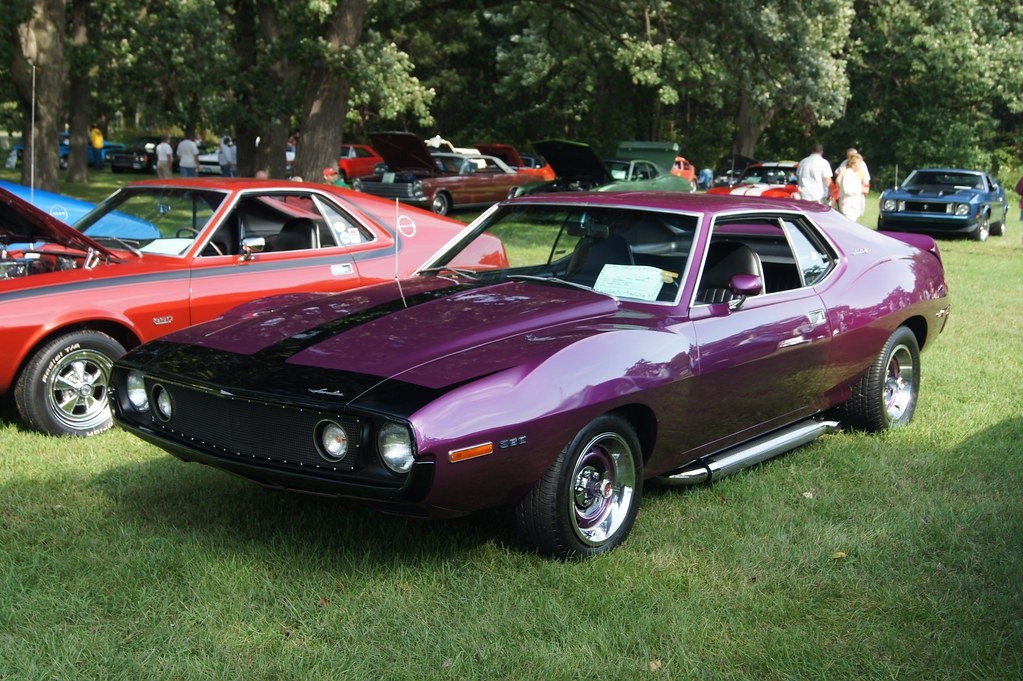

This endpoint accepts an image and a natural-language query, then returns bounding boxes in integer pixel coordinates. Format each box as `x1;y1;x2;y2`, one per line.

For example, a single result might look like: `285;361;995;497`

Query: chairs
562;235;634;286
668;242;765;305
755;174;768;184
622;167;639;182
194;215;239;257
266;218;316;254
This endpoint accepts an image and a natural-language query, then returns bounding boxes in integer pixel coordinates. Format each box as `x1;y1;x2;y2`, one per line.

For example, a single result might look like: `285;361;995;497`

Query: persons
698;158;717;190
287;127;301;177
89;124;104;172
794;142;833;204
222;135;238;179
1016;176;1023;220
155;135;174;215
176;129;206;199
834;148;871;223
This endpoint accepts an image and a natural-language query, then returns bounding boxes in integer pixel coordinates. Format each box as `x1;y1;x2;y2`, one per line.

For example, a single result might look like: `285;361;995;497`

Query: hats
160;133;171;141
323;167;338;175
223;137;231;145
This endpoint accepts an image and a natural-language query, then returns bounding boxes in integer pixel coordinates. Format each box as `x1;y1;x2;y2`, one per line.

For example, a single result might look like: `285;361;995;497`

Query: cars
12;131;125;171
106;189;951;563
877;168;1008;243
530;137;693;195
0;178;163;252
196;134;293;176
339;142;390;184
357;128;546;217
102;137;208;175
424;134;556;185
705;160;839;207
0;176;507;441
671;156;699;193
